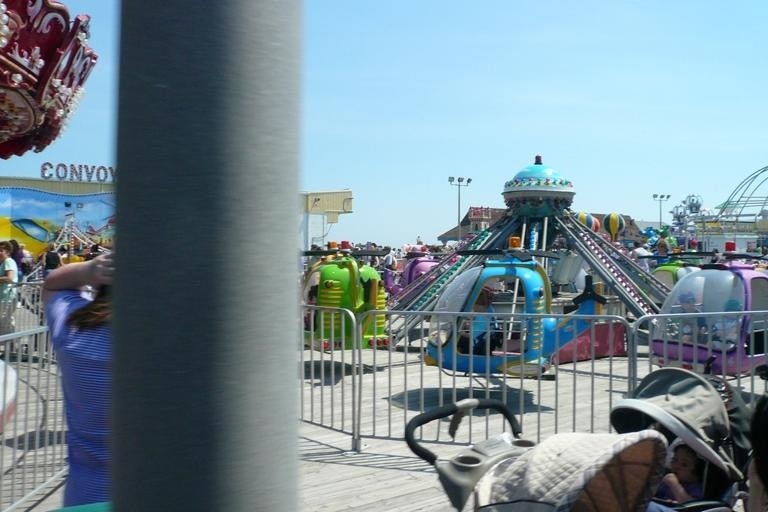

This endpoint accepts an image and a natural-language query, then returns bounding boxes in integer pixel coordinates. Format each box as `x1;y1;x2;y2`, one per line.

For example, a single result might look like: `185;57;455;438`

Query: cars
0;212;86;265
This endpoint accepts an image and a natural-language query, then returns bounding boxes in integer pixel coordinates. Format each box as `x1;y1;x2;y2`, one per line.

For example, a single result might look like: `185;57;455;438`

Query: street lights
652;193;671;228
447;175;474;241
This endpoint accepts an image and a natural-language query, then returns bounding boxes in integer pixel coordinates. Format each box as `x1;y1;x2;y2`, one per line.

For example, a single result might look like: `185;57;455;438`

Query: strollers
602;364;767;512
402;394;672;512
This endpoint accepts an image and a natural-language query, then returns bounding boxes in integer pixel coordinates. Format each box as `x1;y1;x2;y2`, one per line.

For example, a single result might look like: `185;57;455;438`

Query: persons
0;240;33;359
42;241;64;281
370;243;393;292
711;299;743;344
457;286;496;355
671;293;708;340
647;438;707;512
40;251;114;507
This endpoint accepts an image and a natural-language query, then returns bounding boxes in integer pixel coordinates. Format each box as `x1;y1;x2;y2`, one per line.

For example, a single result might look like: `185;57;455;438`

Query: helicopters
635;242;768;380
405;236;605;383
301;247;392;351
